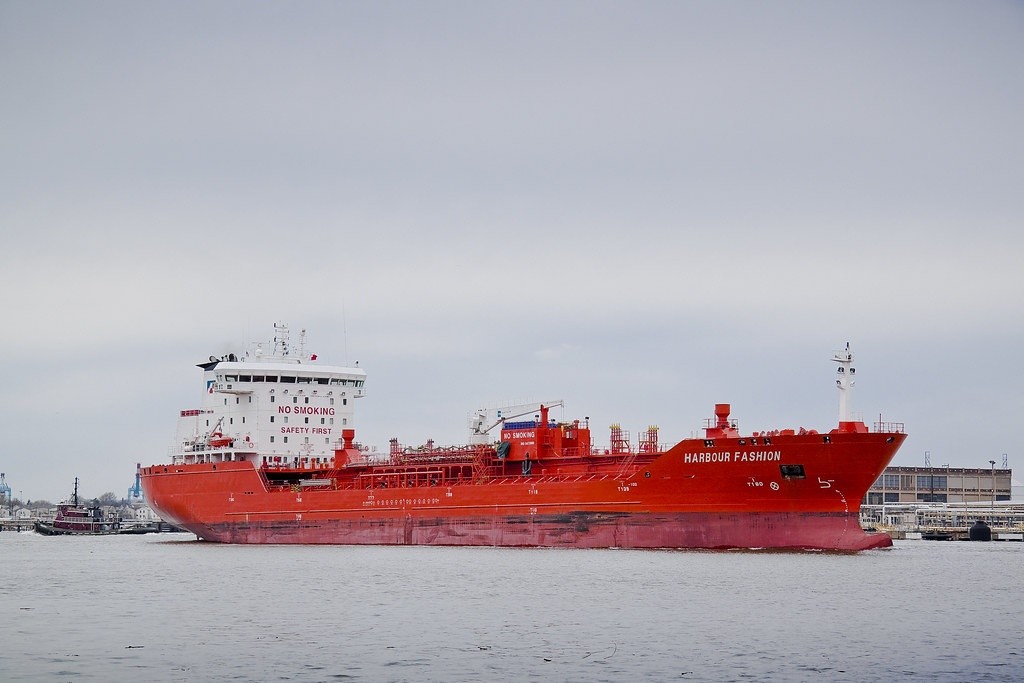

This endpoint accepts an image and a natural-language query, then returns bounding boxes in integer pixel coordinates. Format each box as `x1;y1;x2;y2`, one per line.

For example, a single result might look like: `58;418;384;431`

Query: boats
33;477;120;536
210;432;232;447
137;318;908;556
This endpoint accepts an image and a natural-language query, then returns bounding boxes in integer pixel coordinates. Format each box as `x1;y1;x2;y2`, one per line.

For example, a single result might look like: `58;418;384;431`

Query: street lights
989;461;996;526
20;491;22;508
